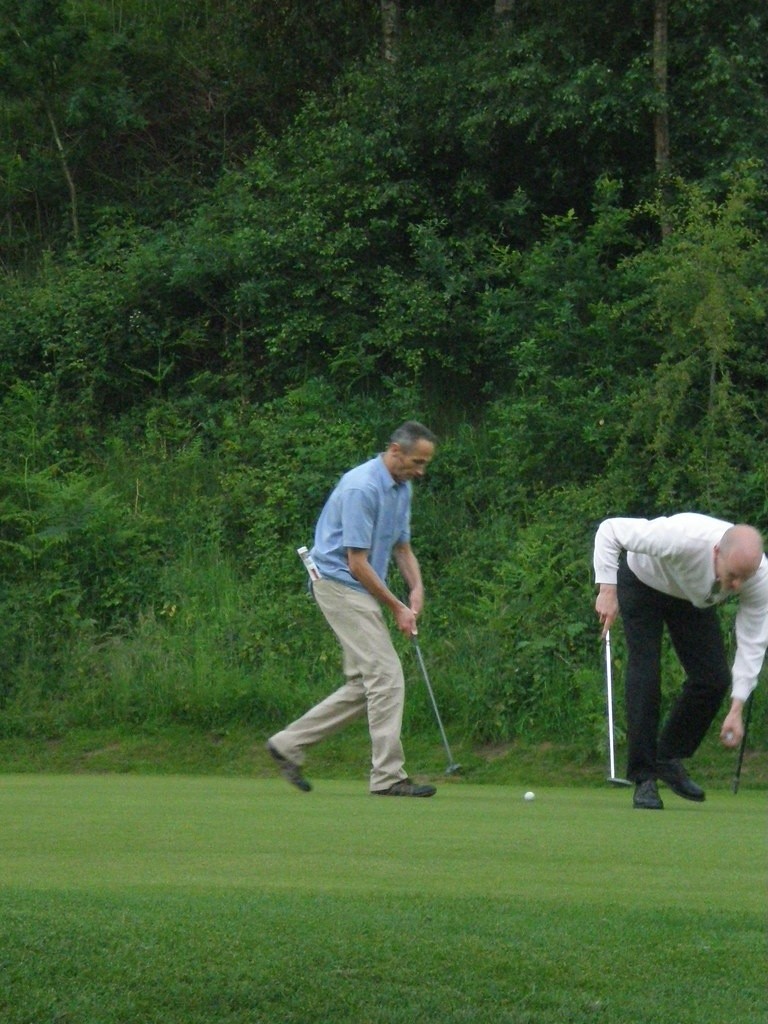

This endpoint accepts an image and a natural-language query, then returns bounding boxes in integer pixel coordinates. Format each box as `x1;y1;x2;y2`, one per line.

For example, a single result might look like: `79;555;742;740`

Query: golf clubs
604;627;635;788
412;621;463;775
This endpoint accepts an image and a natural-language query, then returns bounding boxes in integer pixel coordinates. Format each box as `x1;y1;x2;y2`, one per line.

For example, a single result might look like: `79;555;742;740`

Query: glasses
704;581;722;606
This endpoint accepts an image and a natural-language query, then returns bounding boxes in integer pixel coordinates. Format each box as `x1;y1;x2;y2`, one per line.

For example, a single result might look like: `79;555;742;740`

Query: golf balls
525;791;534;801
727;732;733;740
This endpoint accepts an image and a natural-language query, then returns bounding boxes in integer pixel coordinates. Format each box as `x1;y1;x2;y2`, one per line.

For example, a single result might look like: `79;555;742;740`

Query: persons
593;512;768;810
266;421;437;798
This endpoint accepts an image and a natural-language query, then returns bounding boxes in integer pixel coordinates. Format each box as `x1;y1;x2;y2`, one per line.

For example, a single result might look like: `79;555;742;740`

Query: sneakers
371;779;437;797
264;740;311;791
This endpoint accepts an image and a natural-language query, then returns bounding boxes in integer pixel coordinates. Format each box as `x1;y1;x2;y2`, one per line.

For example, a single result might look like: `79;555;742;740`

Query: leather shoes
655;757;705;801
633;779;663;809
